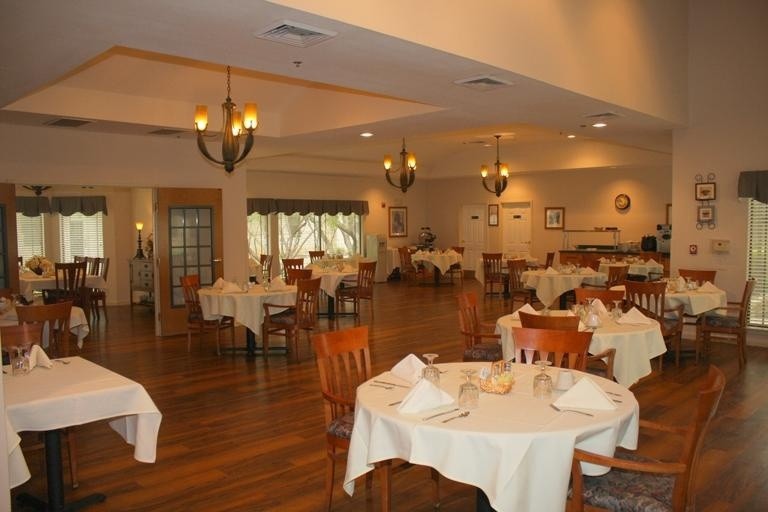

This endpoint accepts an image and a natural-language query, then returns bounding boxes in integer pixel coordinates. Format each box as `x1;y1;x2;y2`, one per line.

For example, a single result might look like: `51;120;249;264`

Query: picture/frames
691;170;718;232
665;202;673;228
544;205;566;232
388;205;409;238
487;203;500;227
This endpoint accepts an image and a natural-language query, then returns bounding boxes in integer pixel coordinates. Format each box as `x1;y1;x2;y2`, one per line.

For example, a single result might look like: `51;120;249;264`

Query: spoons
370;384;393;389
442;411;470;422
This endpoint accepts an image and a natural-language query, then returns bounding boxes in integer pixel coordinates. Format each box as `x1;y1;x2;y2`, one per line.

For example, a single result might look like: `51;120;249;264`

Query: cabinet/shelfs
128;258;155;320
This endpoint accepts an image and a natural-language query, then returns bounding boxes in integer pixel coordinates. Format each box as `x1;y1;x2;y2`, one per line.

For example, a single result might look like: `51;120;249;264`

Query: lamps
383;136;419;194
480;134;512;199
133;220;146;258
193;63;260;174
20;184;54;197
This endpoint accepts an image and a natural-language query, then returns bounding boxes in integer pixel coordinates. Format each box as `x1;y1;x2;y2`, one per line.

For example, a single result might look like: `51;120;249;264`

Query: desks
2;357;161;512
493;310;662;384
18;270;105;307
346;362;640;511
611;286;726;346
196;282;313;356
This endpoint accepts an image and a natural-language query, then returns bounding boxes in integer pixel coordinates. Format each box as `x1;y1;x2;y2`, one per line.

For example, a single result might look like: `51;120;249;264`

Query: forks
549;403;593;417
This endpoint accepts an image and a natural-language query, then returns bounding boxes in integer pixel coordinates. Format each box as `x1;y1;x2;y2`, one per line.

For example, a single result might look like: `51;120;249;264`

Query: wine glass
457;368;479;412
532;360;553;401
422;353;441;387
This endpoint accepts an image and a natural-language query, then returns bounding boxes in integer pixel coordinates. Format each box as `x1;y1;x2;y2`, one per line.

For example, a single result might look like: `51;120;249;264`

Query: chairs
263;274;322;366
624;280;685;373
395;245;667;307
505;327;594;373
662;270;716;350
575;286;626;327
694;280;755;369
455;288;508;361
2;321;81;491
15;299;74;363
311;327;441;511
249;248;378;323
71;256;111;319
54;261;91;335
569;365;725;511
179;275;236;357
518;312;616;380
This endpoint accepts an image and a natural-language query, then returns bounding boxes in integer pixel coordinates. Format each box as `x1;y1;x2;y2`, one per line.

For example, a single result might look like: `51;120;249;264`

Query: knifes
422;407;460;421
373;379;410;388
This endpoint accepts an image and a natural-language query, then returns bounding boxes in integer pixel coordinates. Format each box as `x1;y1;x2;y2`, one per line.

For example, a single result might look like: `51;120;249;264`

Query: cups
7;343;31;377
571;297;624;327
605;255;633;266
554;370;574;391
232;279;269;293
662;276;698;295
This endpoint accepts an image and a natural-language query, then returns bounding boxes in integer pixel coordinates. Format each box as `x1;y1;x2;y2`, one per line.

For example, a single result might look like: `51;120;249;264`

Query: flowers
24;255;54;271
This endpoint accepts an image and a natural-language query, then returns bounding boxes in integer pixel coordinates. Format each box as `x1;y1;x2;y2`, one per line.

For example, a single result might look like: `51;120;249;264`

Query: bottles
490;360;512;384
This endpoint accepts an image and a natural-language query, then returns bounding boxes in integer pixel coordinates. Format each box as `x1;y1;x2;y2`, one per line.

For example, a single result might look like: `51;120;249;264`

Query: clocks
615;193;630;209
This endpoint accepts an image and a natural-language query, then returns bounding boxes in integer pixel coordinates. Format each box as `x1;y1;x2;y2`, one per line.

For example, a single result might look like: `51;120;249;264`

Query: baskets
479;360;515;395
